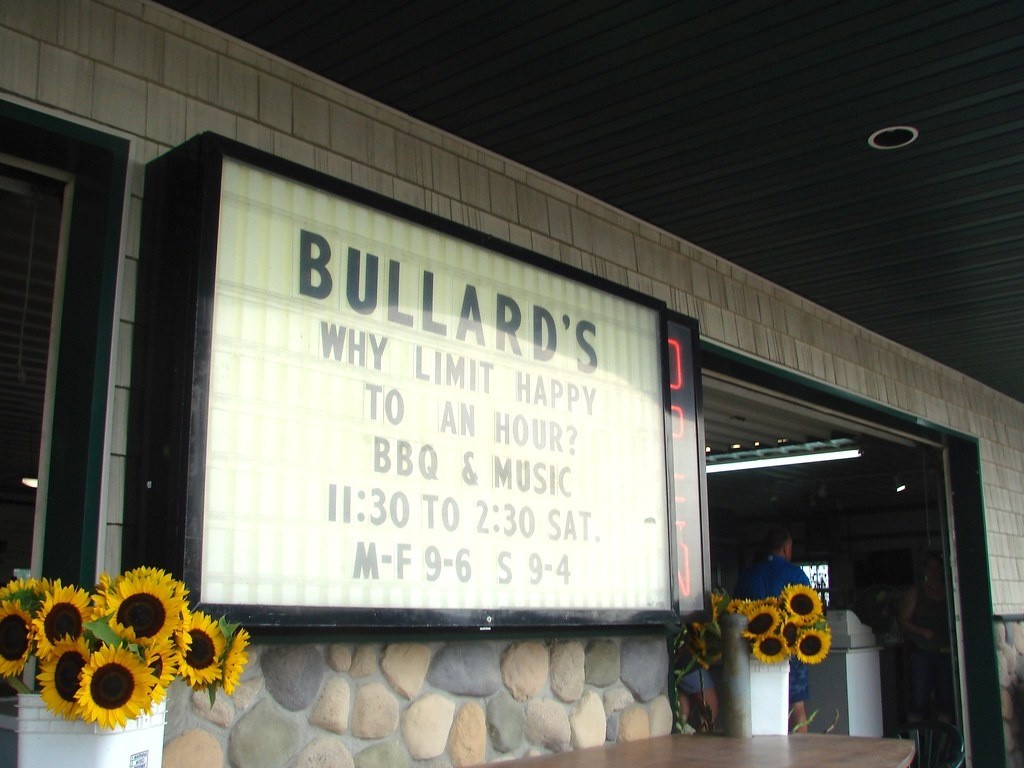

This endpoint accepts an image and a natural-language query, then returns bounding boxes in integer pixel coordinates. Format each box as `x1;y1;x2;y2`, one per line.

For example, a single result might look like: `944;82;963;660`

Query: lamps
706;444;865;473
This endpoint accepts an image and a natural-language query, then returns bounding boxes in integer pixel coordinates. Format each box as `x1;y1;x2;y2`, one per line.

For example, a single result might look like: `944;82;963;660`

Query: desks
470;733;916;768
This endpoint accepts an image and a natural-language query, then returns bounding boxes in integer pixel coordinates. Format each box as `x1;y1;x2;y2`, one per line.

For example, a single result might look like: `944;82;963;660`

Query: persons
740;526;811;733
676;648;719;727
897;551;956;748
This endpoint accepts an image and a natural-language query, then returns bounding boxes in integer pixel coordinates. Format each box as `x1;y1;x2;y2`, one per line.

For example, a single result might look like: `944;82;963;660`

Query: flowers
0;566;251;725
670;585;831;733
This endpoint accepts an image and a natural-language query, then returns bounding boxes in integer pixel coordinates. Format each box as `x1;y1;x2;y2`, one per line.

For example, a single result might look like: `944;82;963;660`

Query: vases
718;613;790;736
18;687;170;768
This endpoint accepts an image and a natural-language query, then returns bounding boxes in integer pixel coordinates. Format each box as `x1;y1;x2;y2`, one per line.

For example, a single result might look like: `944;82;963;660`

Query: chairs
883;720;965;768
